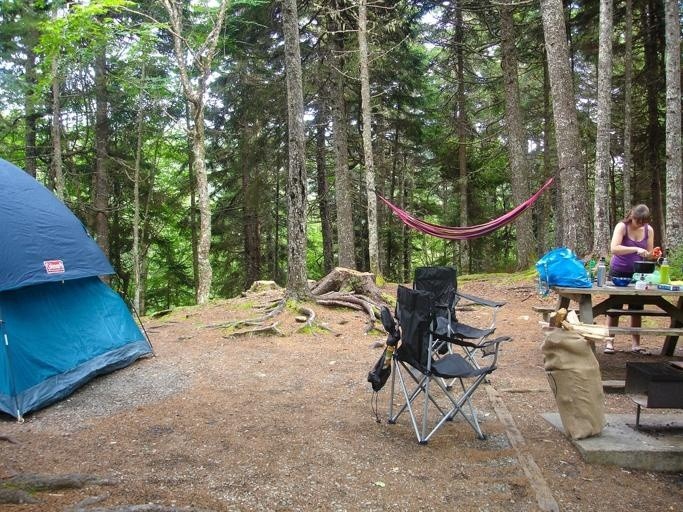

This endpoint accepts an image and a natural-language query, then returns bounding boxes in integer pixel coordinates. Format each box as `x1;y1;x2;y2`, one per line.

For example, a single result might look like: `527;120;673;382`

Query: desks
550;279;683;355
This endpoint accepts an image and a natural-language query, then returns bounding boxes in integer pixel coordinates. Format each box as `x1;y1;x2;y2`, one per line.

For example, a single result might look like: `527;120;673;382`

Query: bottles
659;257;670;283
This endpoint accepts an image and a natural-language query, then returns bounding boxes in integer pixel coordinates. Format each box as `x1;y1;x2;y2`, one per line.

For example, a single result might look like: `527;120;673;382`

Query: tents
0;155;156;421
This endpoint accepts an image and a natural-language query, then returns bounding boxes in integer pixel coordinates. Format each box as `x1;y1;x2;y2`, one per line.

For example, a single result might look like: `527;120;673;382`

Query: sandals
632;346;652;356
604;344;615;354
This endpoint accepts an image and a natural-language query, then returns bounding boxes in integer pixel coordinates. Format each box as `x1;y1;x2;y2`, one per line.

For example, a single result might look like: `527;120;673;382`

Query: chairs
390;285;496;444
409;266;503;389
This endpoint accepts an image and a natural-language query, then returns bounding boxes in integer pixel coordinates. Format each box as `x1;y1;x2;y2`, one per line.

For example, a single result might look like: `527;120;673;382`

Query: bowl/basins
612;277;632;287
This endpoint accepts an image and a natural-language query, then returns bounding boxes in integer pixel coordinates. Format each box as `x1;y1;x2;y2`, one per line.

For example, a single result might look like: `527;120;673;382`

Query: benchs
569;324;683;354
533;301;683;334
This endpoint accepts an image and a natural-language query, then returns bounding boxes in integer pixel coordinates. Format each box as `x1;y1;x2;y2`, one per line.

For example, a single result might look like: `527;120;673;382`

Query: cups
597;265;606;287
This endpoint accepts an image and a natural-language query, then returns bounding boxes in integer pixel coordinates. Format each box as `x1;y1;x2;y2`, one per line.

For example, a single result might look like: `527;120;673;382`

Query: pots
633;260;656;273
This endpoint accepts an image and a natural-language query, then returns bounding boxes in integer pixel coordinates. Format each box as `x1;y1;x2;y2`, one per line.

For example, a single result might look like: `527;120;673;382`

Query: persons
603;204;662;354
